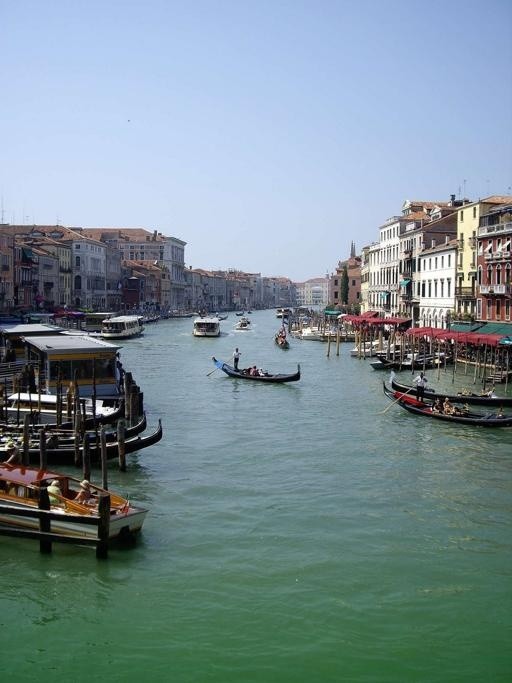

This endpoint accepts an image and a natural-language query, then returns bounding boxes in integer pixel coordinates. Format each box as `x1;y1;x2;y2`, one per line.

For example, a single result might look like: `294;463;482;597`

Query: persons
46;480;62;505
412;372;428;404
233;348;241;371
498;407;504;418
1;481;18;496
4;442;22;466
246;365;266;377
432;398;465;417
276;324;288;345
76;480;91;500
492;383;497;393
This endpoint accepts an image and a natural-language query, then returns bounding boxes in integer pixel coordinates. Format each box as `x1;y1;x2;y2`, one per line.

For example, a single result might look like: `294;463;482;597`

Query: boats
383;376;512;426
193;314;220;337
276;308;292;318
0;462;150;545
234;316;253;330
349;338;454;371
0;310;162;461
274;329;290;350
218;310;252;321
284;307;366;340
212;357;300;382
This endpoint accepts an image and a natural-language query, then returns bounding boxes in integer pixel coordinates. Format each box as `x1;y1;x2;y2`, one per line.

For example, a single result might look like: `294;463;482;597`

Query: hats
51;480;62;489
80;479;90;489
